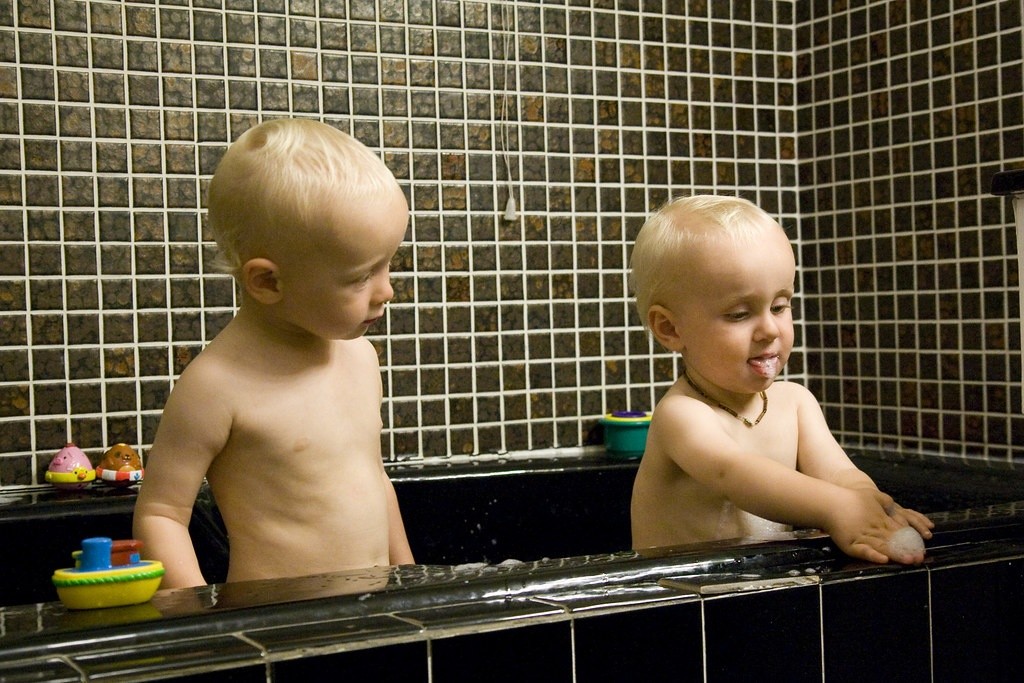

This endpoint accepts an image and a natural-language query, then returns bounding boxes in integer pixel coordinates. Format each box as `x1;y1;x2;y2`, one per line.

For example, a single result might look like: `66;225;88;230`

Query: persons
630;196;936;563
132;118;418;591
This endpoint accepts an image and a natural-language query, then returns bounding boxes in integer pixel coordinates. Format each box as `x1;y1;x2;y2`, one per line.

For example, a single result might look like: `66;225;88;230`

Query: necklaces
683;369;768;427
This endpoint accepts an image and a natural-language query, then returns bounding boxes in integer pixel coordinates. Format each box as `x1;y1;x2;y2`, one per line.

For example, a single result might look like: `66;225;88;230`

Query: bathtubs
0;443;1024;682
